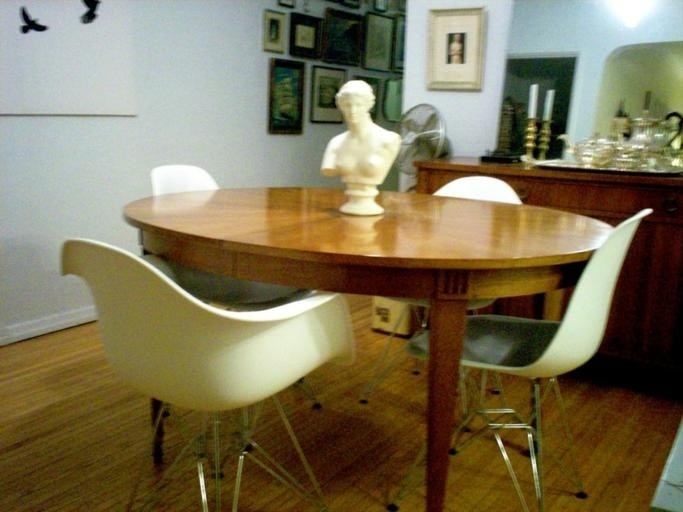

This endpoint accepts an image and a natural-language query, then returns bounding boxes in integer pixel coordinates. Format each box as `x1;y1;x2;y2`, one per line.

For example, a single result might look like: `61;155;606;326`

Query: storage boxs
367;293;420;338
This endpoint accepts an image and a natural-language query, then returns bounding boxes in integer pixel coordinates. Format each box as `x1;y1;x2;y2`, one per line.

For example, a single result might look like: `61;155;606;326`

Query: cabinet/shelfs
410;153;682;387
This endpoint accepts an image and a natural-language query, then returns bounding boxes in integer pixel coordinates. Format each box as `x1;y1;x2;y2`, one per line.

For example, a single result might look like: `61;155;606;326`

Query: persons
319;81;399;185
447;34;462;64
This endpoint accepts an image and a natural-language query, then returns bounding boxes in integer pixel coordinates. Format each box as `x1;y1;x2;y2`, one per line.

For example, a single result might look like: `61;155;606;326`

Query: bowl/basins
611;157;641;170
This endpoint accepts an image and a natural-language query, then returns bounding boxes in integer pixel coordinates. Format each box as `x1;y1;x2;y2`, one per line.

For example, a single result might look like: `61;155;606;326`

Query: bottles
613;97;632;138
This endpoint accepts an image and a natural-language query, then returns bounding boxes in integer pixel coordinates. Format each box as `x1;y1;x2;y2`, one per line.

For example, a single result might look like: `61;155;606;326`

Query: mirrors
494;51;582;163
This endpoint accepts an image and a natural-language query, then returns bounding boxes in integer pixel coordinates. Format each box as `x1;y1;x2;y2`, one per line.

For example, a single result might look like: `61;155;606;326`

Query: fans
390;103;445;193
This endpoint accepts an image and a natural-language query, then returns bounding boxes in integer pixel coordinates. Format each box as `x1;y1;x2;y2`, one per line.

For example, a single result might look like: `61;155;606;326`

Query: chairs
382;206;653;511
149;164;322;472
352;178;527;455
59;239;357;510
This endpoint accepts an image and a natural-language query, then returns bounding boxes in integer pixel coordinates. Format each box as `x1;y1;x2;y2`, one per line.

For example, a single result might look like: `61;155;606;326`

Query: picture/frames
257;0;405;135
423;6;486;95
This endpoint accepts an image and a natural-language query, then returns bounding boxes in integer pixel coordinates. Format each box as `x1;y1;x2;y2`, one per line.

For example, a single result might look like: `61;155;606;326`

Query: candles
541;89;554;119
527;84;538;118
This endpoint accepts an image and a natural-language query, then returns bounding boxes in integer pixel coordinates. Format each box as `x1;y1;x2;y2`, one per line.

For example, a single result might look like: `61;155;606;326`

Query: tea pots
625;107;682;153
553;127;614;167
